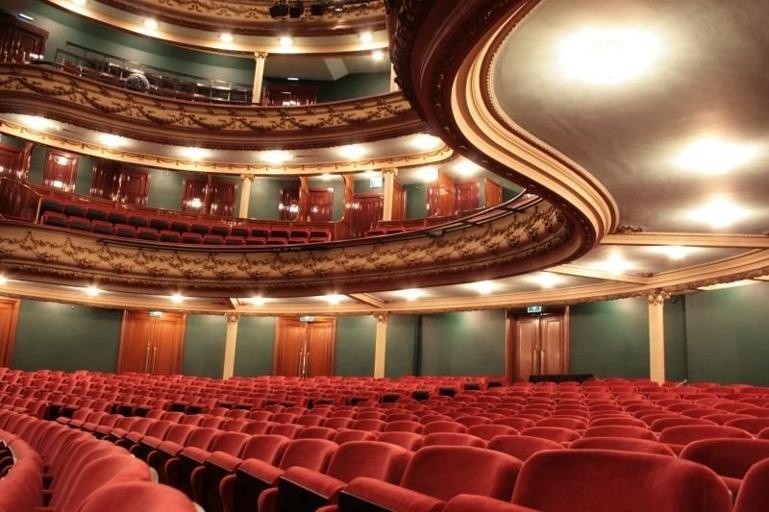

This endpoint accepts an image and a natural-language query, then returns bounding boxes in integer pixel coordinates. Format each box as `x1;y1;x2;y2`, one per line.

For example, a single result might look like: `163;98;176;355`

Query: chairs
36;195;333;244
0;366;769;512
64;58;252;105
363;227;424;236
